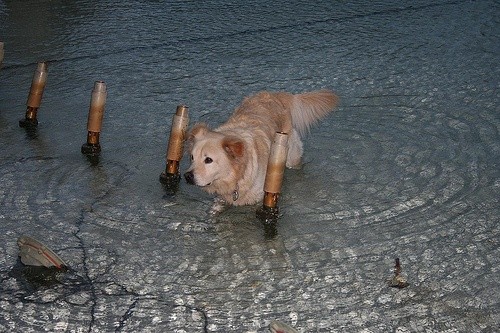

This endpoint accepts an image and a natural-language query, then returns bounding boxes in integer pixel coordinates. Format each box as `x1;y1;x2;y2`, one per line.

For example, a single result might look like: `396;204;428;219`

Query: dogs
183;89;341;215
